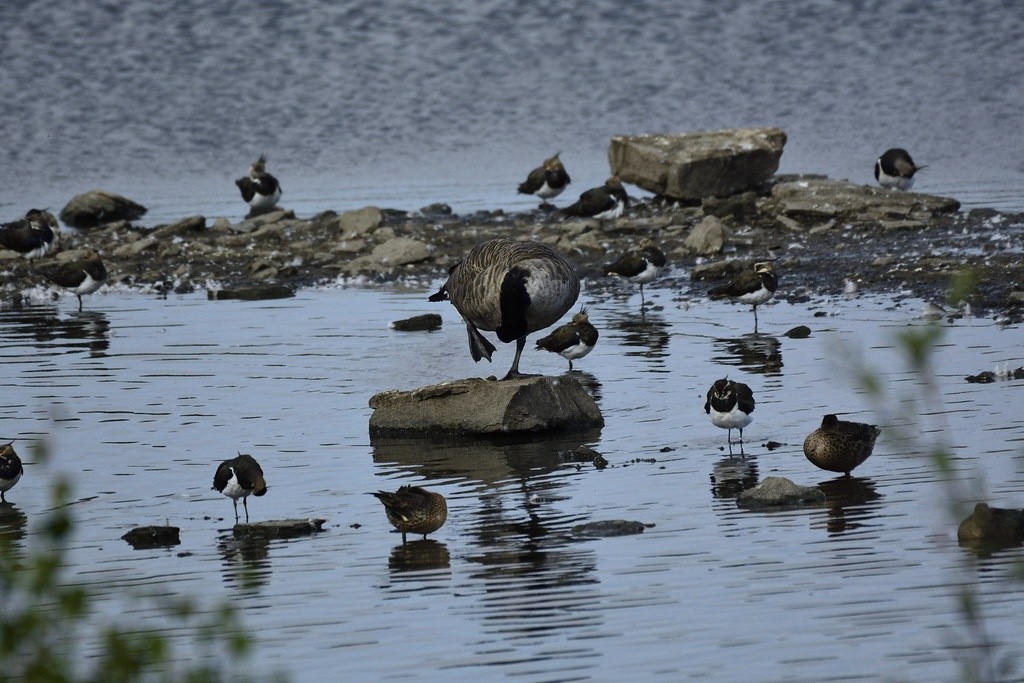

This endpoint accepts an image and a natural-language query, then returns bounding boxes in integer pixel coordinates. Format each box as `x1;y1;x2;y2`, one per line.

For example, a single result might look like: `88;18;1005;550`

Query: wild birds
211;450;268;519
39;240;107;305
0;207;62;272
964;366;1024;384
363;484;447;539
600;236;667;290
517;151;571;207
704;375;756;443
60;190;148;226
559;176;630;227
535;302;599;371
804;415;883;479
957;502;1024;540
874;148;930;192
0;439;25;502
235;152;284;216
427;237;581;382
706;262;778;321
